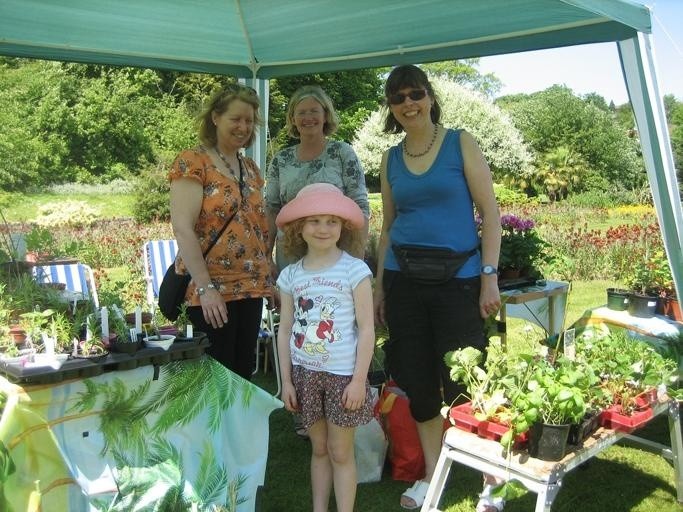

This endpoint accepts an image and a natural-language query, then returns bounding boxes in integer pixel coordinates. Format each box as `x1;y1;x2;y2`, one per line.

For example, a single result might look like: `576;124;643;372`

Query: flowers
476;214;557;269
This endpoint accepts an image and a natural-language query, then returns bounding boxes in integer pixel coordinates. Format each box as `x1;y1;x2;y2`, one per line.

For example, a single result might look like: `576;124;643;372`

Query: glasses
391;90;425;104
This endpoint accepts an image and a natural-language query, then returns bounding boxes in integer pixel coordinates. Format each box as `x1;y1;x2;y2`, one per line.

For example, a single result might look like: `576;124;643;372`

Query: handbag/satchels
353;381;425;483
391;244;477;285
158;262;192;321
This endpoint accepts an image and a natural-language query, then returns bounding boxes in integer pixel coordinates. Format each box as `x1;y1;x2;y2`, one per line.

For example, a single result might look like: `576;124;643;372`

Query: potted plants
443;323;679;461
0;272;207;369
606;245;682;321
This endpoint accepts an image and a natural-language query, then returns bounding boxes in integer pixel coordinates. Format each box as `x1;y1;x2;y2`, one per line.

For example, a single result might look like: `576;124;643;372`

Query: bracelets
198;283;216;296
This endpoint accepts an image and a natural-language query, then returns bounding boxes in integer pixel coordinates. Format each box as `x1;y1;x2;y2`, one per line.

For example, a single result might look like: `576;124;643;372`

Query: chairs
31;262;100;312
141;238;273;374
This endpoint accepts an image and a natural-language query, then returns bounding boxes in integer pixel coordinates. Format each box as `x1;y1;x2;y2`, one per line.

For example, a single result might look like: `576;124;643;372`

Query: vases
504;270;519;280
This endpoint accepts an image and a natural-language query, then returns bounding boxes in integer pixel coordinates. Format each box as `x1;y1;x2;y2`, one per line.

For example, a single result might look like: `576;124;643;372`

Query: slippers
476;485;506;512
400;479;430;509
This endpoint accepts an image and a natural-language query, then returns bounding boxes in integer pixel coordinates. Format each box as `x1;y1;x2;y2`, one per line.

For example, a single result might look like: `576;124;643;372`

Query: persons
372;64;506;512
168;84;281;381
276;182;376;512
265;85;370;271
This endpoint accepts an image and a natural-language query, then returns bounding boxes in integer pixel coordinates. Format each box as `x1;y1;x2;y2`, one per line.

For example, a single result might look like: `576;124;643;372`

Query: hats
275;183;364;232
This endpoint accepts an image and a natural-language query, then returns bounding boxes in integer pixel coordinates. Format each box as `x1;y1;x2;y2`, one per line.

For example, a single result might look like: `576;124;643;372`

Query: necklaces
214;144;247;187
404;122;439;158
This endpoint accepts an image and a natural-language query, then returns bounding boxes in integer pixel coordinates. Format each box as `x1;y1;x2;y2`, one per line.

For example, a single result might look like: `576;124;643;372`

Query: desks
420;401;683;512
0;329;285;512
500;281;569;354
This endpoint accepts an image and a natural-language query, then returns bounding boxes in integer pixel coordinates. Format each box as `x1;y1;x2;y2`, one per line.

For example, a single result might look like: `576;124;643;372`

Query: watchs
480;265;498;274
